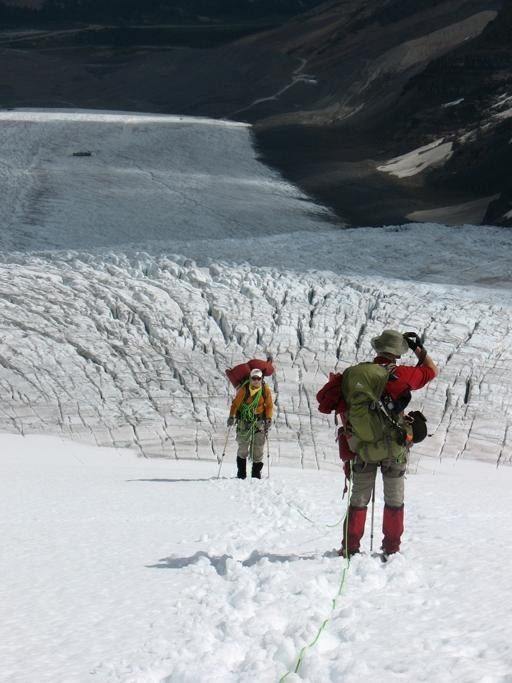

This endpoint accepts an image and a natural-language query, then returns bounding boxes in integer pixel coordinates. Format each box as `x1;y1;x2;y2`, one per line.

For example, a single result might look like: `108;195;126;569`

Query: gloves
266;418;272;429
402;332;423;350
226;417;234;427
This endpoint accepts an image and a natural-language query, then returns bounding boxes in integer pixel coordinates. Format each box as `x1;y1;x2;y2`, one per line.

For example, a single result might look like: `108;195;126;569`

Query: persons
338;328;440;553
227;366;274;481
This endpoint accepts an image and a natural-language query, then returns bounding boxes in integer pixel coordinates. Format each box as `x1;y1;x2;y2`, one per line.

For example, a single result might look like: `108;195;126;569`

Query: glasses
252;377;261;381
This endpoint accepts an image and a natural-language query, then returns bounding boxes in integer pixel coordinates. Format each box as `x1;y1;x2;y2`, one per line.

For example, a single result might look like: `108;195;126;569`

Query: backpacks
225;360;274;388
340;362;408;464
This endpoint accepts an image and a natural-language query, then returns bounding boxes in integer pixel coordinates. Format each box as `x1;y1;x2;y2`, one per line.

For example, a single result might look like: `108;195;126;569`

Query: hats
370;329;409;357
249;368;263;378
